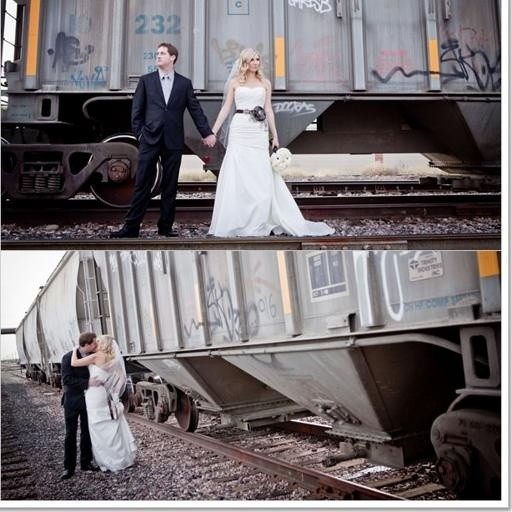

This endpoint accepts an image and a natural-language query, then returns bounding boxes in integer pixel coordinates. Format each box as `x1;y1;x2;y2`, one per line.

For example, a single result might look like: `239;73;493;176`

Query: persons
60;332;98;479
202;46;336;237
111;42;217;237
69;334;139;472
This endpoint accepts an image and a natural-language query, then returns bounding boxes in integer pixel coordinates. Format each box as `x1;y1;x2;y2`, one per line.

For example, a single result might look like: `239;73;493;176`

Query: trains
0;1;501;209
15;249;501;502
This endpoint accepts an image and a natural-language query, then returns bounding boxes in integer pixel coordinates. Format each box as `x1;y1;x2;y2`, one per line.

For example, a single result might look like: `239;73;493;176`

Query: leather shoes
61;462;99;480
109;224;178;238
